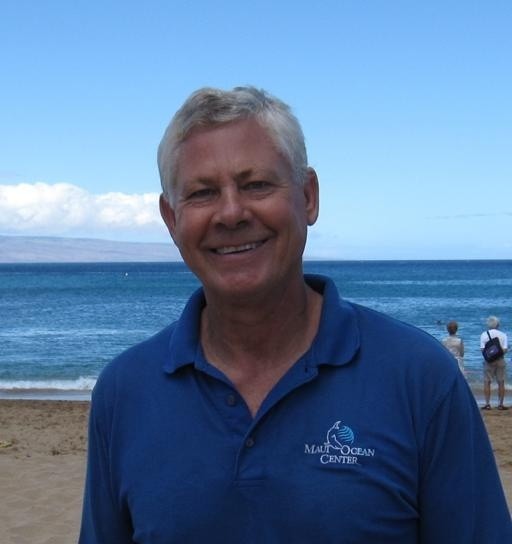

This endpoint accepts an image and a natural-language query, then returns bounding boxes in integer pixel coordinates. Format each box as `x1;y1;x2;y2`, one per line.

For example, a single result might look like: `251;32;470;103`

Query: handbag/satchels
482;331;503;362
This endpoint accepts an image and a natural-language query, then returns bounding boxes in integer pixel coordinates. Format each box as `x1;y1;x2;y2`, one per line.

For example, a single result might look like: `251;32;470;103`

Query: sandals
481;404;508;410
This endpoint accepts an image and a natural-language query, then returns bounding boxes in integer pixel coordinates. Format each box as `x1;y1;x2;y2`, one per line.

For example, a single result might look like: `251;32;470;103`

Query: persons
440;321;467;379
77;84;511;543
480;316;510;411
